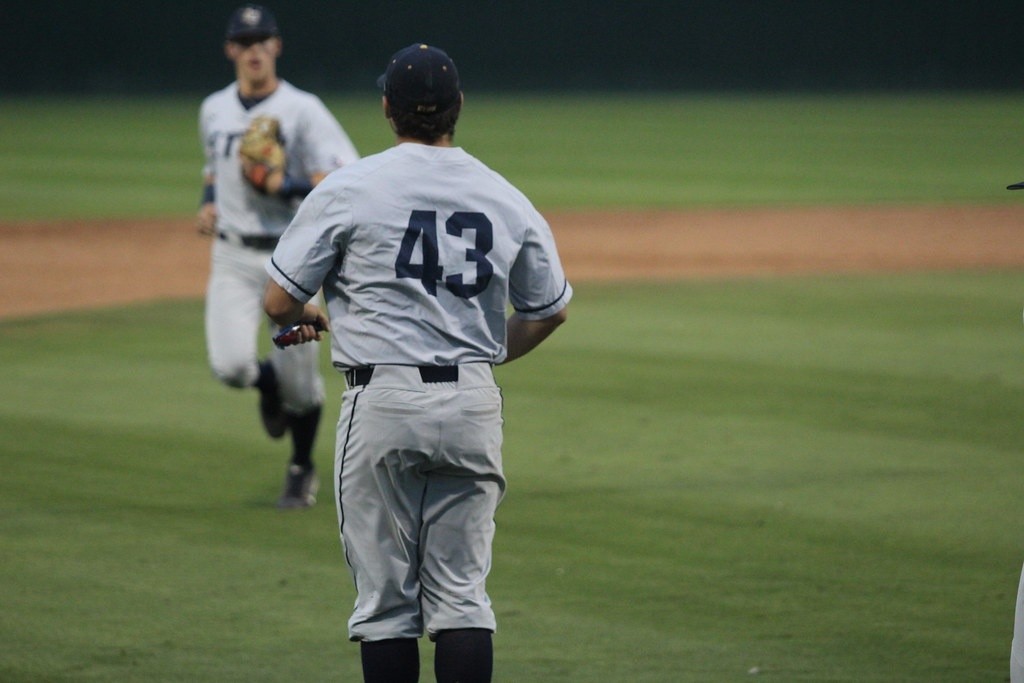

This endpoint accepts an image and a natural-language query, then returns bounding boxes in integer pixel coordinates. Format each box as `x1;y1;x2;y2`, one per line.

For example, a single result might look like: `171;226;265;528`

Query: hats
377;43;461;113
225;5;278;38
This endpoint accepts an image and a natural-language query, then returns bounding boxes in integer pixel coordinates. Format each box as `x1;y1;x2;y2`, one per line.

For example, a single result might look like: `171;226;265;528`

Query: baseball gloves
237;116;288;193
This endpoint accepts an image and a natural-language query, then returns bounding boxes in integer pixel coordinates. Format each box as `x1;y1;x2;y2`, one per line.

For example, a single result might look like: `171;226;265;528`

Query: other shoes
258;361;288;437
278;462;318;508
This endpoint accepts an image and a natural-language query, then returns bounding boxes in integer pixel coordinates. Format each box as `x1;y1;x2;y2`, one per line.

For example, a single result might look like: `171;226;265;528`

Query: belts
218;230;279;251
346;358;459;387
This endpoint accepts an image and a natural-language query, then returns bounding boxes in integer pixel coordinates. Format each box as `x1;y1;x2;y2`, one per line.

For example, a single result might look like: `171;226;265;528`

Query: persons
193;6;362;514
262;42;573;683
